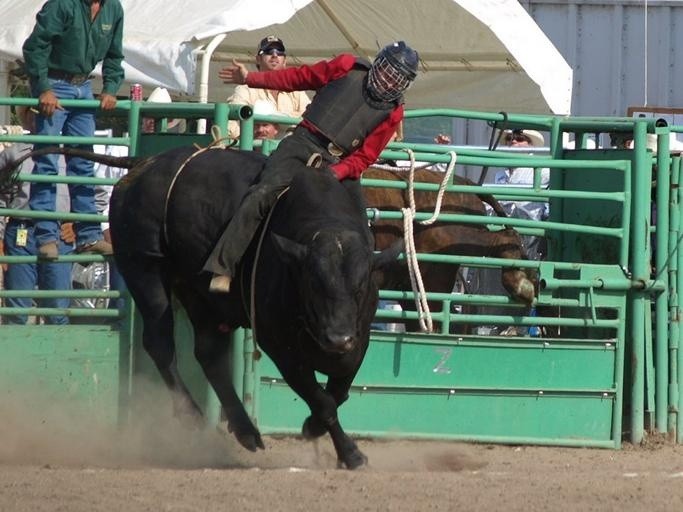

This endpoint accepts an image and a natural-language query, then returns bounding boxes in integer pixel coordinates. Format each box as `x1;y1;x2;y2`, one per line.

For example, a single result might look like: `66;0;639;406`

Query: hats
252;100;290;140
146;87;181;130
258;35;284;49
496;127;545;147
630;130;683;155
566;138;603;150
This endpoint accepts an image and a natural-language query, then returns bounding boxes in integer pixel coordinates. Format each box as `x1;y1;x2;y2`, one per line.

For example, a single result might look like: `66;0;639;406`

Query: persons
226;34;315;140
608;128;633;151
252;99;294;141
94;86;186;324
379;127;450;176
0;95;76;325
493;128;551;336
202;40;420;295
21;1;127;263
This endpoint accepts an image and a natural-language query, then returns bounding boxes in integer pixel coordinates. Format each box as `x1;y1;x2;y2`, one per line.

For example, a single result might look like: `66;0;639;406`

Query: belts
48;67;96;84
325;140;347;160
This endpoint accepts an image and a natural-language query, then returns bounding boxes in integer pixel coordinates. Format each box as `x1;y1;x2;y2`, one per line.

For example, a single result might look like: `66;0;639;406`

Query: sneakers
39;243;59;260
76;241;113;255
209;272;232;292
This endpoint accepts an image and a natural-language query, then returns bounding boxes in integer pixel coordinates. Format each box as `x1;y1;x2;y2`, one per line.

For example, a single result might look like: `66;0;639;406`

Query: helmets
368;41;419;102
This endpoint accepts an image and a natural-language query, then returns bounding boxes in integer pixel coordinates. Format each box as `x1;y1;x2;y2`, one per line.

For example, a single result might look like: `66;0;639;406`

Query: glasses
507;135;530;142
259;50;285;56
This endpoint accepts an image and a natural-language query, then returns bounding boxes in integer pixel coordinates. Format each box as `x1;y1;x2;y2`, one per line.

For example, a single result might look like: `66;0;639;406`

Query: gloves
0;241;8;273
60;223;76;245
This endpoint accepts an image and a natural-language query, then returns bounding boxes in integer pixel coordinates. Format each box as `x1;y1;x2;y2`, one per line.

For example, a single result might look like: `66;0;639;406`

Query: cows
0;145;406;470
359;167;571;337
